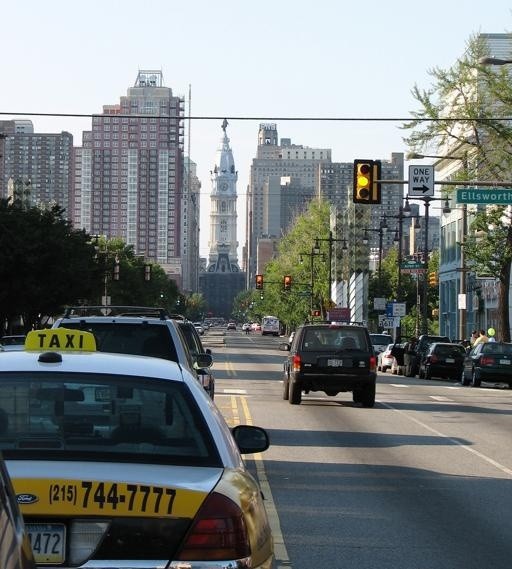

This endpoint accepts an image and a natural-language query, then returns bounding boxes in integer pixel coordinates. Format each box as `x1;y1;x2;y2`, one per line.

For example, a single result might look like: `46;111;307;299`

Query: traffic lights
284;276;291;290
352;160;372;202
256;275;263;289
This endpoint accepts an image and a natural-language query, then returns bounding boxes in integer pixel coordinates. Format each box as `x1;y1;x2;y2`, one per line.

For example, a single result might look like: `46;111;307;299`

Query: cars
192;318;260;335
368;334;512;388
0;326;272;569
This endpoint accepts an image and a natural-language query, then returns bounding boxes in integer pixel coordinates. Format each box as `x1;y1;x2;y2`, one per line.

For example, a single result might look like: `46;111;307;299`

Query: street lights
406;152;468;339
362;196;451;333
298;231;348;309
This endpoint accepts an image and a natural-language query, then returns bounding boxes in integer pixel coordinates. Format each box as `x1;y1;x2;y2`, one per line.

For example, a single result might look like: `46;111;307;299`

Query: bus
261;316;279;335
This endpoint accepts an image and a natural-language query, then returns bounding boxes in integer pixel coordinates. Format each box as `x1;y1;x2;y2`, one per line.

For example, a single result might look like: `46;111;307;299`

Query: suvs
26;305;213;442
279;320;376;404
98;313;215;401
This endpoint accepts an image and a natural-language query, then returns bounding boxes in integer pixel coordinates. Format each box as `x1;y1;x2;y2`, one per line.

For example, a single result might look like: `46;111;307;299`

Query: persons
470;330;488;348
403;337;420;377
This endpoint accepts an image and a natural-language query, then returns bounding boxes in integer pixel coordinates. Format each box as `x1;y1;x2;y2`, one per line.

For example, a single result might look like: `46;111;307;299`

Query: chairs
341;338;356;350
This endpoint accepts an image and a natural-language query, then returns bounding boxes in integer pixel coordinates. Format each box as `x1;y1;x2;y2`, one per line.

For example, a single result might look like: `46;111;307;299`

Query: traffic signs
409;164;434;196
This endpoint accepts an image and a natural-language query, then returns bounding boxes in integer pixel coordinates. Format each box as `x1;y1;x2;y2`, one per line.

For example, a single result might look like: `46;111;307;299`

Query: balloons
488;328;495;342
